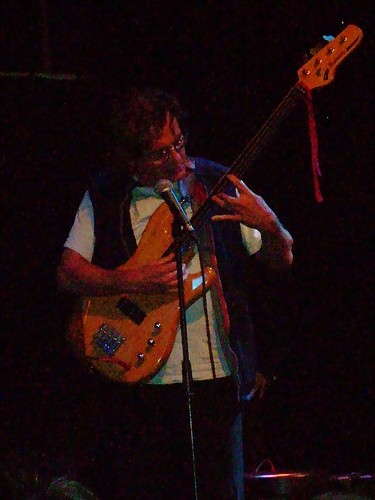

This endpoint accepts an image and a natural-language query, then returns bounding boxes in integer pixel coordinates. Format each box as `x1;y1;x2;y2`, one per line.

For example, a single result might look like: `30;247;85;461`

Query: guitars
62;20;365;390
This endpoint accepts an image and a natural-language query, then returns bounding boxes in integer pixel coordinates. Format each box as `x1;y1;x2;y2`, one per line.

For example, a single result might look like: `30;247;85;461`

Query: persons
58;86;293;500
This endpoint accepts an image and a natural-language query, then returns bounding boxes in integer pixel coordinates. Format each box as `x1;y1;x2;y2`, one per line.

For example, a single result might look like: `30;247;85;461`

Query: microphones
154;179;199;244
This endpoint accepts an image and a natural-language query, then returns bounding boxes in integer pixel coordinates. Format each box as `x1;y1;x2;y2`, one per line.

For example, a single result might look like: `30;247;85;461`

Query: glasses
142;128;192;164
